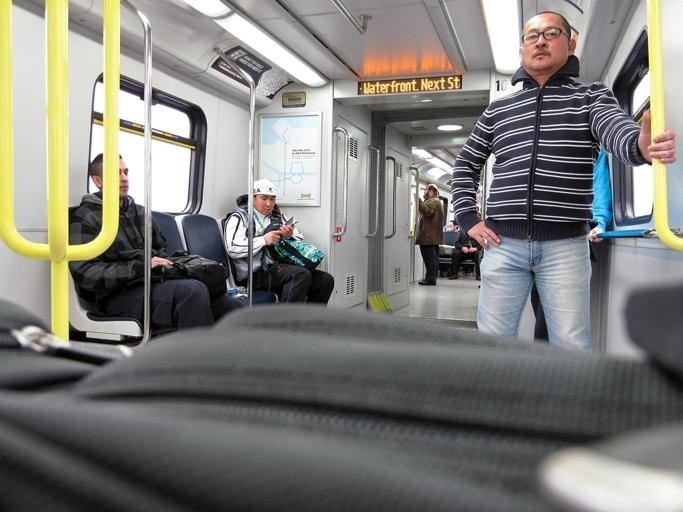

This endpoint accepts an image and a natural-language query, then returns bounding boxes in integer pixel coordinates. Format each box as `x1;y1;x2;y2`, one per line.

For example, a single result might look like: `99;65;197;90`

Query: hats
254;180;278;197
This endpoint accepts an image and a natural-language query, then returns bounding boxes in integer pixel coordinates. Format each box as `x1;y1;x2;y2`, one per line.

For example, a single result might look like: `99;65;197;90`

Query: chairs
438;231;481;278
68;206;297;346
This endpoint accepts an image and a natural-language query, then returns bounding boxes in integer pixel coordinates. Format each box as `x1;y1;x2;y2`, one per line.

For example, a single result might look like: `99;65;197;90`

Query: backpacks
265;223;325;268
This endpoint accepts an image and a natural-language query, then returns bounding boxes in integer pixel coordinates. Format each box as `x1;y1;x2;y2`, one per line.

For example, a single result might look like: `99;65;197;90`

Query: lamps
478;0;523;76
179;0;329;89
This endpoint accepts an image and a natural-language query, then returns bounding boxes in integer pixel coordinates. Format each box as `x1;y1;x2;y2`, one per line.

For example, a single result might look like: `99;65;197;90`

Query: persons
531;143;613;340
69;150;245;330
437;219;482;280
452;12;676;352
225;180;334;303
414;184;444;286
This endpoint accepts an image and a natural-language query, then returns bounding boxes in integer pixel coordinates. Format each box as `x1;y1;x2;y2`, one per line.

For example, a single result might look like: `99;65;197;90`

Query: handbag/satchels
153;247;226;284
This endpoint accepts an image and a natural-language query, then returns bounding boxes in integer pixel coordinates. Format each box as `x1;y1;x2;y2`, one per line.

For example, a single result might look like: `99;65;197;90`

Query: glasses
521;28;571;45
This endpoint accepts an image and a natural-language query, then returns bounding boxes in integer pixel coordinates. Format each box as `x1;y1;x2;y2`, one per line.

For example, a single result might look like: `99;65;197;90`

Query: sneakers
476;275;482;280
451;274;458;279
419;278;436;286
